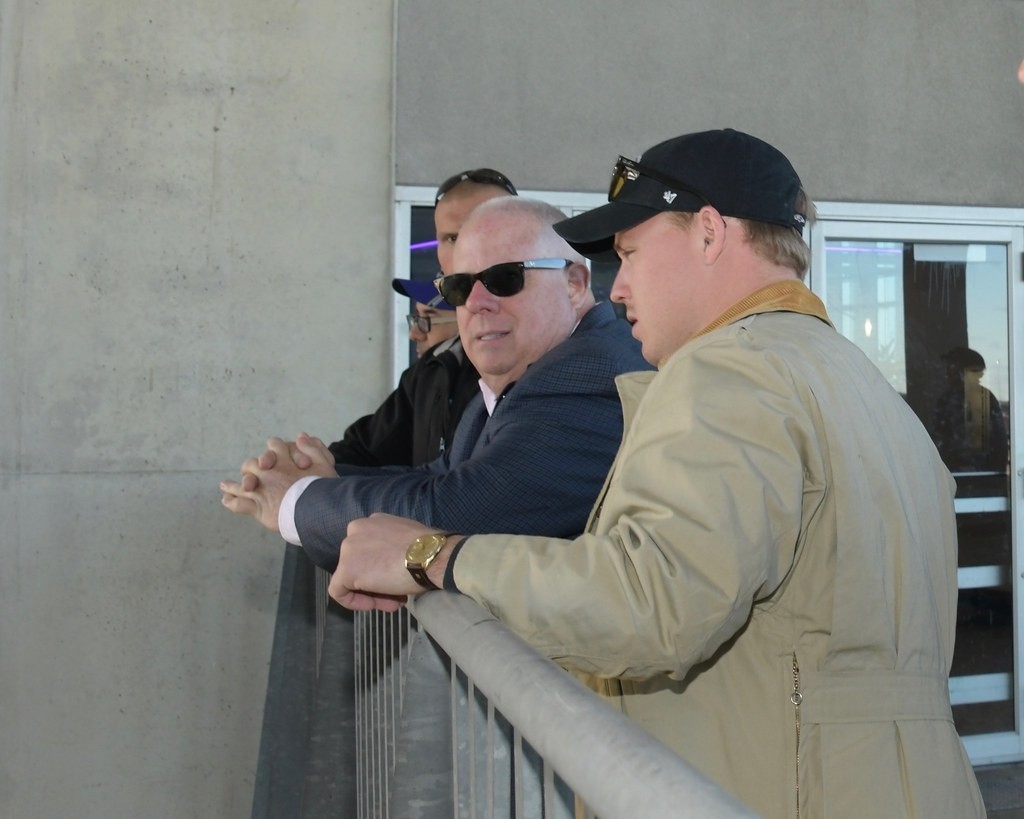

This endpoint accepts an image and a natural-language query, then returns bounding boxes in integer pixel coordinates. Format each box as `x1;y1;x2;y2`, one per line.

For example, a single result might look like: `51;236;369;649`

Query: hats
392;270;457;311
553;129;806;263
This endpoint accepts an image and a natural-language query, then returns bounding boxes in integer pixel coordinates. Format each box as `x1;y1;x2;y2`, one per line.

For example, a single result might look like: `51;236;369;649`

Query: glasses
433;258;574;307
608;154;727;227
406;315;457;334
435;168;518;209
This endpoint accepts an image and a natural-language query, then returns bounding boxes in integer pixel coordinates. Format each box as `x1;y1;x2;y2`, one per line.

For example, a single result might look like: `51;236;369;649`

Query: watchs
405;527;467;591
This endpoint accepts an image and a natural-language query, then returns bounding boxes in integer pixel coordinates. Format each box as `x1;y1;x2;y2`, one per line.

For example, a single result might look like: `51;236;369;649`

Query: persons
218;168;661;574
328;125;989;819
